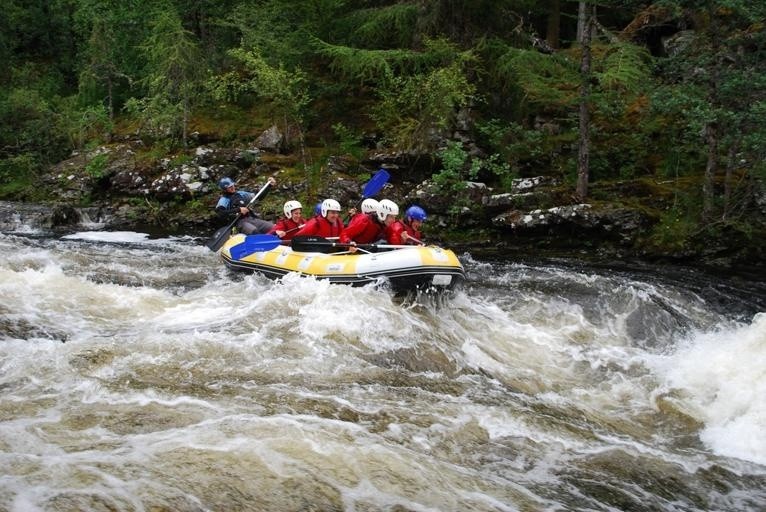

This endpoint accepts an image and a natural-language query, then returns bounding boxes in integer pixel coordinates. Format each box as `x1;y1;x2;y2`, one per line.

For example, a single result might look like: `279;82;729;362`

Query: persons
215;177;277;235
293;198;359;240
347;198;379;227
339;198;409;255
266;199;309;246
397;204;428;246
312;201;324;217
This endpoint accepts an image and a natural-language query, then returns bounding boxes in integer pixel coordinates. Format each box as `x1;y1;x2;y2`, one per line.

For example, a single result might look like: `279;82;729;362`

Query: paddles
207;182;271;252
291;235;418;252
230;223;308;260
342;169;390;223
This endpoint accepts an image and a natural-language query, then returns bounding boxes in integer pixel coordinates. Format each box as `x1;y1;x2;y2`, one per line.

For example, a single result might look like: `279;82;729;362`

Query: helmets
283;200;303;220
314;202;323;216
404;204;427;224
320;199;341;218
360;198;379;216
376;198;400;222
219;176;235;190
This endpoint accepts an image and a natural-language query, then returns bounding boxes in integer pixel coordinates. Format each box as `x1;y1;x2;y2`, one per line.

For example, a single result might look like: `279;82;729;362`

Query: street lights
220;232;467;296
